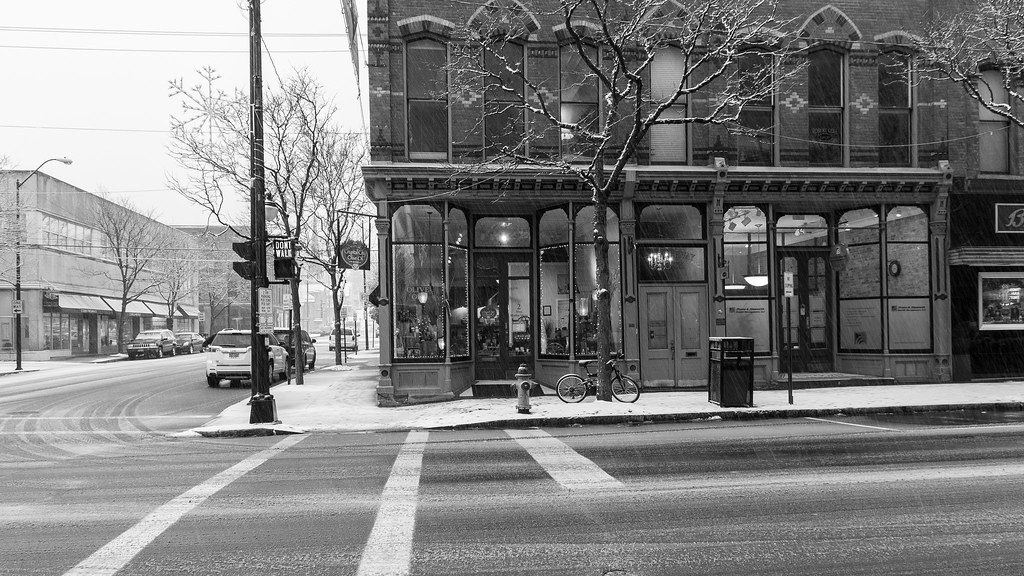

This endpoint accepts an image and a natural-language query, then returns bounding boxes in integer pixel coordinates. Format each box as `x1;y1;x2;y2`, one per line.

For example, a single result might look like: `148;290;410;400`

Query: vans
273;326;317;372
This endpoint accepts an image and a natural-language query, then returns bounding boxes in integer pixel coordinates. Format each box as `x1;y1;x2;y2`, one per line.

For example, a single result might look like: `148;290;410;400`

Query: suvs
205;327;289;388
127;329;177;360
329;328;360;351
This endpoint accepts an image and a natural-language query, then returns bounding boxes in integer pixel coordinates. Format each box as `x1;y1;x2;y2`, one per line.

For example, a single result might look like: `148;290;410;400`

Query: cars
174;332;206;354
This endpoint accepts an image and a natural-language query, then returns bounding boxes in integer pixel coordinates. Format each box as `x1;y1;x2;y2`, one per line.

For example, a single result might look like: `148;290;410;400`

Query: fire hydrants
510;363;539;413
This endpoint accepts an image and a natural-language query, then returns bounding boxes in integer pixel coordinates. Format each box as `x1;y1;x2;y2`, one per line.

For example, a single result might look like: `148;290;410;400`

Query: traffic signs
12;300;23;314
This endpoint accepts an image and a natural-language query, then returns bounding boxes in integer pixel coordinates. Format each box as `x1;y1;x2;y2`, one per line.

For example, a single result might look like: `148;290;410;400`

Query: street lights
14;156;74;371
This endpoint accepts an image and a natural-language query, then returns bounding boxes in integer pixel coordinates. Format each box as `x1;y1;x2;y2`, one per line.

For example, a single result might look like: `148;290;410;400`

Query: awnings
42;291;201;319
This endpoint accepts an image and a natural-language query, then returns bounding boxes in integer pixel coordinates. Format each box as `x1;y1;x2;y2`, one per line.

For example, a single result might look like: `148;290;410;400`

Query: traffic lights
231;240;258;279
273;258;297;281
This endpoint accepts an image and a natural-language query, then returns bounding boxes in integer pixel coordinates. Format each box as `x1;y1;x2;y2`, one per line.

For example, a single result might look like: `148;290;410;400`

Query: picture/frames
977;271;1024;330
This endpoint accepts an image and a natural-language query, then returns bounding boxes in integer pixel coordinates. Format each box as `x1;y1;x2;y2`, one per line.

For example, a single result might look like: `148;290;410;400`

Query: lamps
743;223;768;286
741;215;751;227
647;248;675;271
728;218;736;231
725;243;745;290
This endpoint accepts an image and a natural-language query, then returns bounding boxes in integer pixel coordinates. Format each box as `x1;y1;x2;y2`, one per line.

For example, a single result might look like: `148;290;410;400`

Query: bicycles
555;351;640;404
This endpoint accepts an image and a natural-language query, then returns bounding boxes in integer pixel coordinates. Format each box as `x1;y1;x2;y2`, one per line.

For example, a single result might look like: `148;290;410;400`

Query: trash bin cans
708;336;755;408
